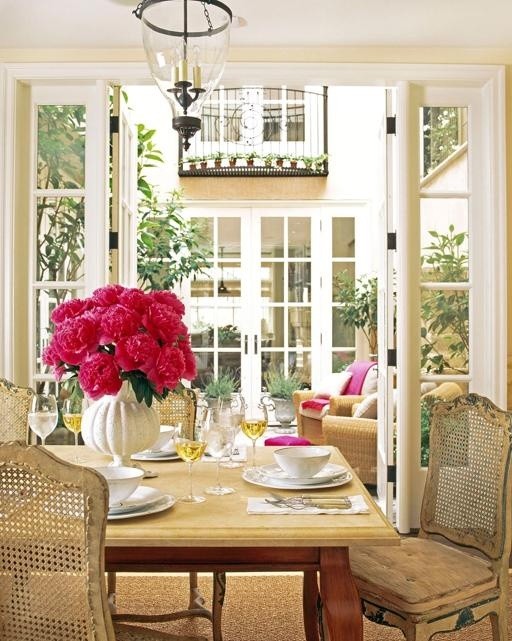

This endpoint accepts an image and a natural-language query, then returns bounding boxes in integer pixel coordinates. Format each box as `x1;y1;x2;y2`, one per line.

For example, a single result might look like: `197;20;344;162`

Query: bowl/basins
274;445;330;481
145;425;176;454
92;466;145;508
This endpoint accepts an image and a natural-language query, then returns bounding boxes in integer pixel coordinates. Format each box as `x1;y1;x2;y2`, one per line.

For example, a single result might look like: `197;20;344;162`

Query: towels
246;492;371;518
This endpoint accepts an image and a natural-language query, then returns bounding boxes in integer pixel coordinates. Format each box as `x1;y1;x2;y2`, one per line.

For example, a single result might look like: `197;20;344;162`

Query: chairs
312;389;512;641
106;382;235;641
288;358;396;448
316;381;464;490
1;378;36;619
1;438;209;641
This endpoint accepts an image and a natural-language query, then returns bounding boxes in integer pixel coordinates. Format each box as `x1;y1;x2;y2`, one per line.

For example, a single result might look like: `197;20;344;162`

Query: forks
262;492;354;511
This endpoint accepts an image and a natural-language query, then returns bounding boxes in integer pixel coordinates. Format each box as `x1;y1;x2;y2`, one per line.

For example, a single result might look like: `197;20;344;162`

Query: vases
74;375;164;479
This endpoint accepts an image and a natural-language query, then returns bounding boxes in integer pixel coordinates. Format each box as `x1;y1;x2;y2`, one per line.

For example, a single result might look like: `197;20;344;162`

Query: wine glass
62;398;89;463
29;394;59;448
200;396;268;498
174;421;207;506
259;395;296;435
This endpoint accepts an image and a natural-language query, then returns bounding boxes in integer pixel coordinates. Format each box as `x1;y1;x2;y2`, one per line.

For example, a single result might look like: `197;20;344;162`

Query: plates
131;440;181;464
108;484;164;515
108;494;175;522
244;465;352;492
260;466;348;486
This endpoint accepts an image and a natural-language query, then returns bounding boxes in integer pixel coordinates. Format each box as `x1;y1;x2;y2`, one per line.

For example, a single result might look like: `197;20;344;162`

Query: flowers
40;282;199;407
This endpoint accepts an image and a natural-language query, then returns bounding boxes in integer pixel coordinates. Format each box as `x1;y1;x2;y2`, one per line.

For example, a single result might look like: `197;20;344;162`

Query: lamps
218;247;231;294
131;1;238;153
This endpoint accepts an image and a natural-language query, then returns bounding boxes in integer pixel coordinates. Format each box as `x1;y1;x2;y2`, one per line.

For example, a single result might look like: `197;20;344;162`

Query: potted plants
191;361;245;424
263;359;313;436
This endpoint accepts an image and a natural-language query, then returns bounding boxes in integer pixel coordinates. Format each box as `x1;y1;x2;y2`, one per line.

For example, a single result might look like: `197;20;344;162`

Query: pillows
350;390;379;422
313;371;353;402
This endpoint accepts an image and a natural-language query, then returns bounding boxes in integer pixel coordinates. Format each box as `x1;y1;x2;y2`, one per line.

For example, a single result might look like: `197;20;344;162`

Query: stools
263;435;312;447
